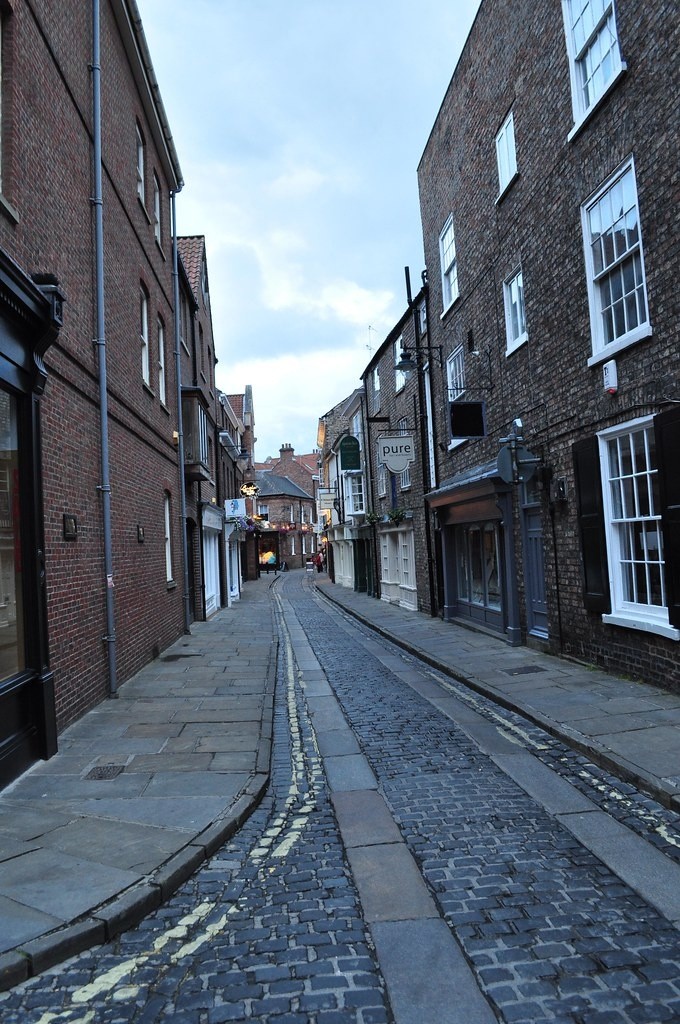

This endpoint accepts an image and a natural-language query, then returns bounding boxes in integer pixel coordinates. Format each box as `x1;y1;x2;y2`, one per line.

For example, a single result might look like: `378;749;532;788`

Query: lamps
393;344;443;372
222;444;251;460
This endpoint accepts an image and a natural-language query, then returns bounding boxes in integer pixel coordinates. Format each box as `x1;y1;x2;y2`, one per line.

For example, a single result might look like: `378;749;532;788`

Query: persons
310;551;327;573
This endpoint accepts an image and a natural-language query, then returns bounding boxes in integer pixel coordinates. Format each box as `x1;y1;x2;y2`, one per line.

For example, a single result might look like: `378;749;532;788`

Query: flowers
279;527;289;536
240;517;270;533
